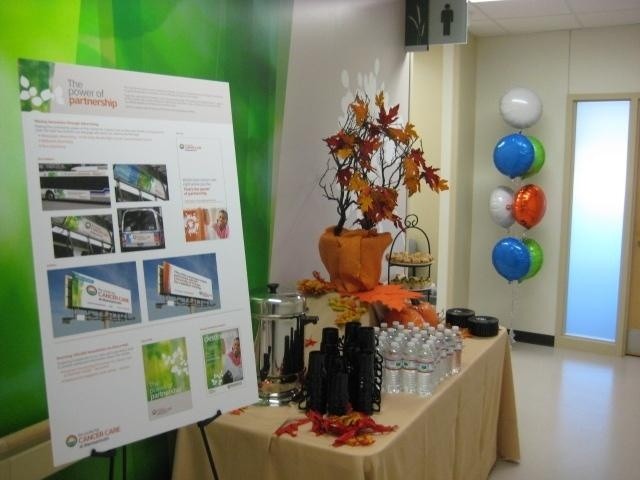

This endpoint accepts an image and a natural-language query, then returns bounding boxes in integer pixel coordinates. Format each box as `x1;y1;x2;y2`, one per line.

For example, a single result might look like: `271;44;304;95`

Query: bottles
368;321;462;396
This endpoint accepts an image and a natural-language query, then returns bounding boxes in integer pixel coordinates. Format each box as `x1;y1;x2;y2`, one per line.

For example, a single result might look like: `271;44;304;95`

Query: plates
382;279;436;292
446;308;499;337
387;253;435;267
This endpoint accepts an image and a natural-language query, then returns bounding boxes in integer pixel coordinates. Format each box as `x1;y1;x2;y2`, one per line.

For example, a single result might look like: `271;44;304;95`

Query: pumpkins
381;298;440;329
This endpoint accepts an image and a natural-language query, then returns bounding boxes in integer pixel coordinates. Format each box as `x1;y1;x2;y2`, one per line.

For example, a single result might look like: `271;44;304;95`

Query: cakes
392;273;432;289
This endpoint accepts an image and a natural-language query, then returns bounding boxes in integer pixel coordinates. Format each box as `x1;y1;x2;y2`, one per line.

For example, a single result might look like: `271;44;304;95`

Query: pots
248;282;319;406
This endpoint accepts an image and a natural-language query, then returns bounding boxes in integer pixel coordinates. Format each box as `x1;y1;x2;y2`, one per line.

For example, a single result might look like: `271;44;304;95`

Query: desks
180;326;508;480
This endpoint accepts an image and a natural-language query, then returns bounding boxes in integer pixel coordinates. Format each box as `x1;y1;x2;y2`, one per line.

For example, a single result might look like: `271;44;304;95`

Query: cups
299;320;382;417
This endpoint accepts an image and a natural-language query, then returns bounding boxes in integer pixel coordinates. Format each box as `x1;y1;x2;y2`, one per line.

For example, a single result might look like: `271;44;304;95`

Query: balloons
489;87;546;284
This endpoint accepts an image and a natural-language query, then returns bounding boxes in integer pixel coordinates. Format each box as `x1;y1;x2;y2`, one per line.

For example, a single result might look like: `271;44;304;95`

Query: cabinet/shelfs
385;213;431;304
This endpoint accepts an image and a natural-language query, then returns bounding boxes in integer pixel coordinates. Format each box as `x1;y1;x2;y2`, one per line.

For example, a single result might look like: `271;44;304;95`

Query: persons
221;337;243;385
211;209;230;238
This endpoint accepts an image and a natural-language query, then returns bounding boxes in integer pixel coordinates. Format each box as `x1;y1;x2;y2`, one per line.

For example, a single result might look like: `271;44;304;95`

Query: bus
40;170;111;204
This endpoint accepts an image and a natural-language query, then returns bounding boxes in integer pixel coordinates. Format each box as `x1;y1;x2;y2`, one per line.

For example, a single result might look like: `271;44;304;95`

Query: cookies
387;250;435;264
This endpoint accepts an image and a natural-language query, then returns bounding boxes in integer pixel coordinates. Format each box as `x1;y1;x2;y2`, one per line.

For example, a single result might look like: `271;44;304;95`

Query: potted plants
320;84;449;296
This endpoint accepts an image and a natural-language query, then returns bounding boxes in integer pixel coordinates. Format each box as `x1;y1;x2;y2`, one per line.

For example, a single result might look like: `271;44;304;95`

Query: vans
122;208;165;248
